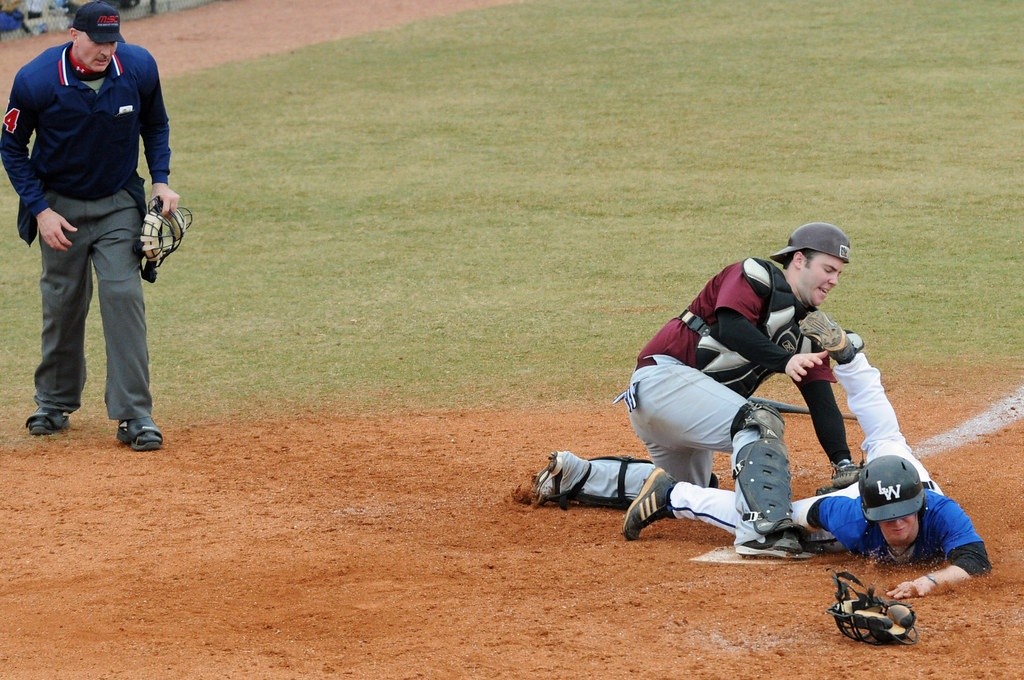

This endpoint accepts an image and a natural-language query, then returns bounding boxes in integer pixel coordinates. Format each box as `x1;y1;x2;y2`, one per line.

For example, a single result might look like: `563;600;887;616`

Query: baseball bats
748;396;857;420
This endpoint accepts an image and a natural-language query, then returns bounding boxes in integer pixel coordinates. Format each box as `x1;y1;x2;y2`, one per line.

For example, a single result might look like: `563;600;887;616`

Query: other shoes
25;407;70;436
116;416;164;451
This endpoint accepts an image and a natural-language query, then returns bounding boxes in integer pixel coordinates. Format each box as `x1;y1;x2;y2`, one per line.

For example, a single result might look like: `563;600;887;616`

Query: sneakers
531;448;567;506
622;466;678;541
736;531;821;559
798;309;855;363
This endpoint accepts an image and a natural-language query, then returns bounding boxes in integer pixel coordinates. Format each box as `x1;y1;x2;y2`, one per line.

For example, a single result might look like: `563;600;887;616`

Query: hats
72;2;127;47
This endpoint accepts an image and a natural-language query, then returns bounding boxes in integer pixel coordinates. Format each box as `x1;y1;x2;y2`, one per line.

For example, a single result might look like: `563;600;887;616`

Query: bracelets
926;575;938;585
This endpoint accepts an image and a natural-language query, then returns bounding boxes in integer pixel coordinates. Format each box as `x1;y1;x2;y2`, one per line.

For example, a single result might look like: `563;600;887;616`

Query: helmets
769;222;852;265
860;455;926;522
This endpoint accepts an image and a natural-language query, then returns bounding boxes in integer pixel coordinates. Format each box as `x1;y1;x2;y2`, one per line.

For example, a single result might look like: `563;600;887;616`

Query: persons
0;1;179;451
532;223;992;598
